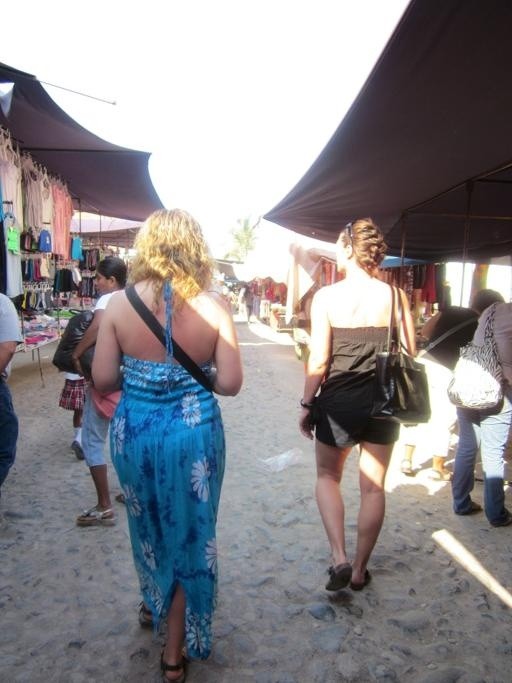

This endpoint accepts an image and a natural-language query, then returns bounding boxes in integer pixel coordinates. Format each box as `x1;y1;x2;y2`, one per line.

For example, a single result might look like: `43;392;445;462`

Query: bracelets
299;397;319;409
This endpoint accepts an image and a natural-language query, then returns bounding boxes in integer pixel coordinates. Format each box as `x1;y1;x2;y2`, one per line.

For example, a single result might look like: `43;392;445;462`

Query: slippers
325;563;352;591
350;569;370;591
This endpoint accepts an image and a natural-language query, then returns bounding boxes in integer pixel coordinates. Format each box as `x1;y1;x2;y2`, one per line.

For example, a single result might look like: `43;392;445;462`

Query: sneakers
491;517;511;527
469;501;481;512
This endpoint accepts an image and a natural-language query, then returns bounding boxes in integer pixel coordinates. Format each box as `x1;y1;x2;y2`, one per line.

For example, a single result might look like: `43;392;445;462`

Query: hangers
4;128;70;198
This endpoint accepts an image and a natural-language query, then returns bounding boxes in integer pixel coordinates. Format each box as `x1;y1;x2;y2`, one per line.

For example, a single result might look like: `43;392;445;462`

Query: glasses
346;223;353;244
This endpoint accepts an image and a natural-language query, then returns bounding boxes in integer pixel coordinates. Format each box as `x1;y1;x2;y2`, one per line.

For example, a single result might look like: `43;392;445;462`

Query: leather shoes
72;441;84;460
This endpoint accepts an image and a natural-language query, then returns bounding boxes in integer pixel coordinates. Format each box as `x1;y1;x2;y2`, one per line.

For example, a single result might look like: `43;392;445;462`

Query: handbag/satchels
369;349;431;423
52;309;95;375
447;343;505;410
89;385;122;421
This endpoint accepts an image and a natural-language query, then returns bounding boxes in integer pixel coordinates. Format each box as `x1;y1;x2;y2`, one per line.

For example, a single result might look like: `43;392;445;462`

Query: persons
56;371;95;460
241;285;255;324
297;216;418;592
400;286;505;483
0;292;22;485
90;208;241;682
70;257;130;527
448;297;512;526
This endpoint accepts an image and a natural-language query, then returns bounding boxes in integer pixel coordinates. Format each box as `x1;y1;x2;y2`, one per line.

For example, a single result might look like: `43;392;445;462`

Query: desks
24;305;93;388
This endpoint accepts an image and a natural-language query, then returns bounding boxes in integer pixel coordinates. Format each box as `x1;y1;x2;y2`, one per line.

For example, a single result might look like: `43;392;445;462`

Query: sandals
77;508;116;526
401;460;413;474
139;603;153;626
433;469;453;481
161;650;186;683
